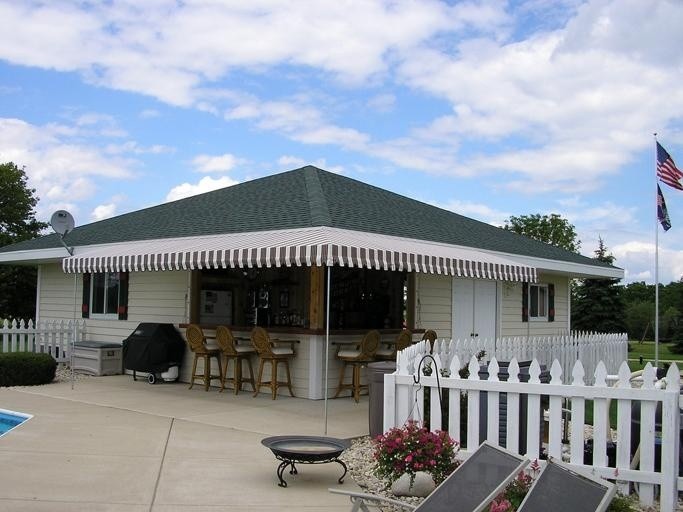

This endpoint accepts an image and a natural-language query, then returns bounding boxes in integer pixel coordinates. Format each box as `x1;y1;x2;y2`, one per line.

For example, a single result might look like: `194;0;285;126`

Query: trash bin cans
367;361;397;442
122;323;185;384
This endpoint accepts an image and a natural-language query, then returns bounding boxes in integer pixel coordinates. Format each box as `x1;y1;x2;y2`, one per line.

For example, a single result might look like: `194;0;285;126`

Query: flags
656;183;671;233
655;141;683;192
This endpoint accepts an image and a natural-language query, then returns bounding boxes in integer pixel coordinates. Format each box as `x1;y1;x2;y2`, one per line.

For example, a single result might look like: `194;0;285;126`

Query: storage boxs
69;340;123;376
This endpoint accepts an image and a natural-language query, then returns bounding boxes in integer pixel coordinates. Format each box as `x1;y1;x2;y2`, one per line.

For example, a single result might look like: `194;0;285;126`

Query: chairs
327;440;619;512
186;323;301;400
331;330;437;403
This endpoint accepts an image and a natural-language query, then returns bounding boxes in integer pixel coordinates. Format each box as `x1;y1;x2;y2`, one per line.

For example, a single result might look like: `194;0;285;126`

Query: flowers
369;420;461;493
